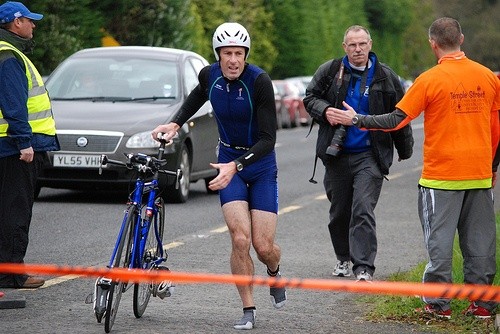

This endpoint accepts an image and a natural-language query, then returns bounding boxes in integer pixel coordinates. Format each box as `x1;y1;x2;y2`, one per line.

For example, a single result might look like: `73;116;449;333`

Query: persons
152;21;288;330
303;24;414;282
325;16;497;320
0;1;61;287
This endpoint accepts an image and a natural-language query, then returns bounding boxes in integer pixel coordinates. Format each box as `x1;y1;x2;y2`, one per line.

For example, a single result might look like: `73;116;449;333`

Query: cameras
320;125;347;165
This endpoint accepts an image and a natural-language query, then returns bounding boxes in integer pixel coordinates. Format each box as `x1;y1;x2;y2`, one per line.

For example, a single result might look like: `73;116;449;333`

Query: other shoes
269;272;287;309
234;310;256;329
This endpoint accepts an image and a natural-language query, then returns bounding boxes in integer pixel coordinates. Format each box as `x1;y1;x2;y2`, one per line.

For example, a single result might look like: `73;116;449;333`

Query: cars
270;75;315;129
30;46;221;202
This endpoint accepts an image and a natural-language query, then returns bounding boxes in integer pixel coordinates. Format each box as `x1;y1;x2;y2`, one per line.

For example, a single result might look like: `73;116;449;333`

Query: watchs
351;114;359;127
233;159;244;172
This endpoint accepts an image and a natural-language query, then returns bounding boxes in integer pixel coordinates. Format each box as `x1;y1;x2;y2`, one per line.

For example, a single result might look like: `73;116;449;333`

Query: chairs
140;80;165;97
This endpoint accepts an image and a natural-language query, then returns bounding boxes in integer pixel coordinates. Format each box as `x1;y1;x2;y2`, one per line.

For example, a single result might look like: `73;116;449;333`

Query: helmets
212;22;250;61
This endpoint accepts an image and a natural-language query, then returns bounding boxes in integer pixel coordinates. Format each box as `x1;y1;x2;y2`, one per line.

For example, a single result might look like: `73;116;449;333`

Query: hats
0;2;44;24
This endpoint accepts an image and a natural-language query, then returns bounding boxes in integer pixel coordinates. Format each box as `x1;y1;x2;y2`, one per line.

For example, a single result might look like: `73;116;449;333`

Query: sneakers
414;305;451;320
332;260;352;277
462;301;492;318
23;277;45;288
356;270;373;281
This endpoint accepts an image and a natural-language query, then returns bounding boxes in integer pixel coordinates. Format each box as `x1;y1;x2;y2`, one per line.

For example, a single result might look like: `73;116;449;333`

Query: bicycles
85;137;183;334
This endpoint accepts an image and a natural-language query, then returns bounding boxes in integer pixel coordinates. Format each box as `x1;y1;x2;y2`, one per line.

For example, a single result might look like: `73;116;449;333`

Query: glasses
345;39;370;49
